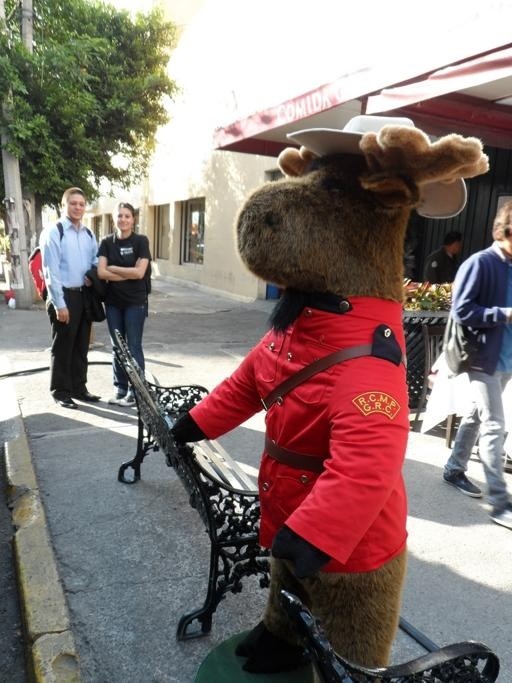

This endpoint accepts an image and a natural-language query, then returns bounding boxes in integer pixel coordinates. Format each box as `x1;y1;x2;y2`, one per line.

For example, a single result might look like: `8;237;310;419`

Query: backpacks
28;222;92;303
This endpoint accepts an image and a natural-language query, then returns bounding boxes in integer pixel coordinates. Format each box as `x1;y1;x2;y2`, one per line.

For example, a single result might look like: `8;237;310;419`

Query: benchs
110;324;273;641
278;584;500;683
410;321;458;449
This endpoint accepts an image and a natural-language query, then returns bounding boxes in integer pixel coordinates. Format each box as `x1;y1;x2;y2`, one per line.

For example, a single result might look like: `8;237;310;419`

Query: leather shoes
72;392;101;401
51;392;78;408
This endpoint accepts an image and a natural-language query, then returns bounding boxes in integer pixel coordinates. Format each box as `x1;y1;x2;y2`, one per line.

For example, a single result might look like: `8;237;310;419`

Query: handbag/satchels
443;317;478;375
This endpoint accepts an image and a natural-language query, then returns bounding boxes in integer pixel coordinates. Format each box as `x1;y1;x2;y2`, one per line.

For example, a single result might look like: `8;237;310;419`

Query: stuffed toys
164;113;486;682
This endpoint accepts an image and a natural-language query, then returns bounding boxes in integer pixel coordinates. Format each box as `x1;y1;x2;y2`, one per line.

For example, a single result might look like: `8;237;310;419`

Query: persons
422;231;463;285
39;186;101;410
441;199;511;530
97;203;151;405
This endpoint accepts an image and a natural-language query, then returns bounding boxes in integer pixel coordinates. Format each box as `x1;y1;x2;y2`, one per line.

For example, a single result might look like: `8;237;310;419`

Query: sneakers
488;502;512;528
106;393;141;407
442;472;482;497
